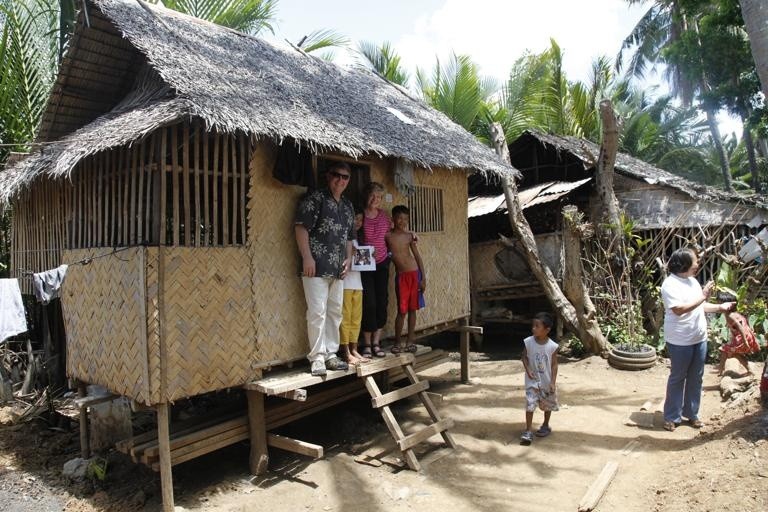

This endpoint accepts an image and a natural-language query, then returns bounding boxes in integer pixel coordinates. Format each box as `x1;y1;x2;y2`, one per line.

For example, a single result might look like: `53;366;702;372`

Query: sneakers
663;417;703;431
311;361;327;376
328;358;348;370
521;427;551;442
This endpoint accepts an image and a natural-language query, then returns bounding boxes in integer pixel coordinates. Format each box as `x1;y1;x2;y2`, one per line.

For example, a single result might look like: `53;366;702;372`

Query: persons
385;205;426;353
354;250;370;265
661;248;736;431
361;182;391;359
520;312;559;441
715;293;761;377
294;163;356;372
341;207;370;356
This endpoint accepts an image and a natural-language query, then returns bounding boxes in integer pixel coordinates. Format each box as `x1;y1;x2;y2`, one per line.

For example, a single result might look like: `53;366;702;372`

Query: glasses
331;171;349;181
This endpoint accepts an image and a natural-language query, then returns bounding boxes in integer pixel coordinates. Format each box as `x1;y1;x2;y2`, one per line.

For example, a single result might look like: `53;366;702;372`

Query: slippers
391;345;417;353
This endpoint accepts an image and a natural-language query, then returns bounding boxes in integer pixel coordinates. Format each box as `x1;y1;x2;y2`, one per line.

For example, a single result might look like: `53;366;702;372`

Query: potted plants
600;210;657;371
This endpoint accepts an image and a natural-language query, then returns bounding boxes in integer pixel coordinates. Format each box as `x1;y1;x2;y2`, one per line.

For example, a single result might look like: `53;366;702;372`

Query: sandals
362;344;386;358
